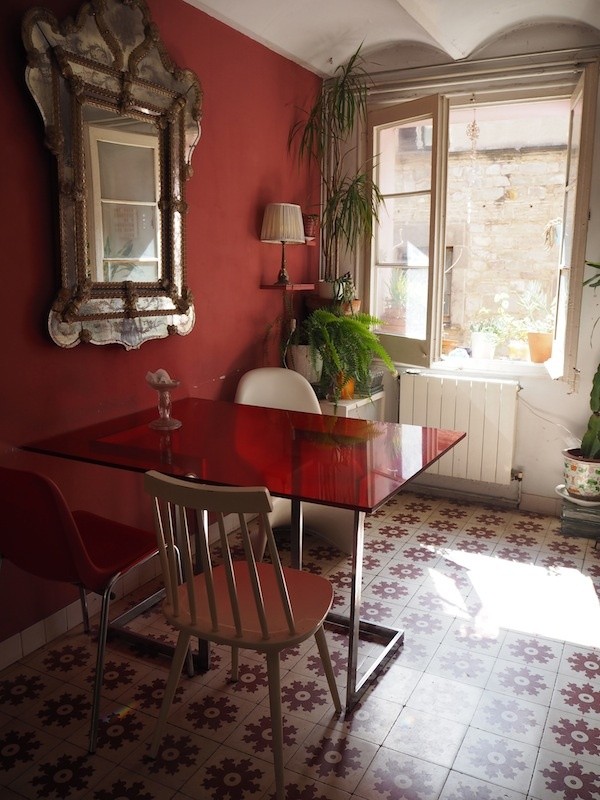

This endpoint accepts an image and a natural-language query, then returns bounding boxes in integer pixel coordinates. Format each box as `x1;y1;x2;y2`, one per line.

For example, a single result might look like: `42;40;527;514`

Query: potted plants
288;36;397;415
555;364;600;508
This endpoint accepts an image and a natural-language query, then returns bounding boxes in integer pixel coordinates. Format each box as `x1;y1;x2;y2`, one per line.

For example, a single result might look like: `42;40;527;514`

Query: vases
528;332;552;363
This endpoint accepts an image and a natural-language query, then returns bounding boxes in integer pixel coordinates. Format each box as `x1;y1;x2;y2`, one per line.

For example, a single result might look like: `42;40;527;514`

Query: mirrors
16;0;202;351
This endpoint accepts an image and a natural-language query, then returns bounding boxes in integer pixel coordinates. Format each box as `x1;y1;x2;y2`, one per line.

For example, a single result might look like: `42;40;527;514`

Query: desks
17;397;466;712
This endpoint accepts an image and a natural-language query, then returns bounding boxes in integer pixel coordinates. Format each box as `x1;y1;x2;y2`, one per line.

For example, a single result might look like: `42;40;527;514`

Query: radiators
398;369;524;485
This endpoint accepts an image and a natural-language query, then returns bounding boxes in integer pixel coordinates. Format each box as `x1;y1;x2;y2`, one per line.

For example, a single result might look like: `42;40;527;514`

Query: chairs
0;466;191;751
144;469;341;800
235;368;354;563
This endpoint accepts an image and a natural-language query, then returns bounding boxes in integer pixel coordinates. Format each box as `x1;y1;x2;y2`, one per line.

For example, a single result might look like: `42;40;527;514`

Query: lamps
260;202;305;285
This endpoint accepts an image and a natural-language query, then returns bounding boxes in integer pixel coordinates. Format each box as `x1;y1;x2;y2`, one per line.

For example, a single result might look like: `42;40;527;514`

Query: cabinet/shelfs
259;242;316;289
